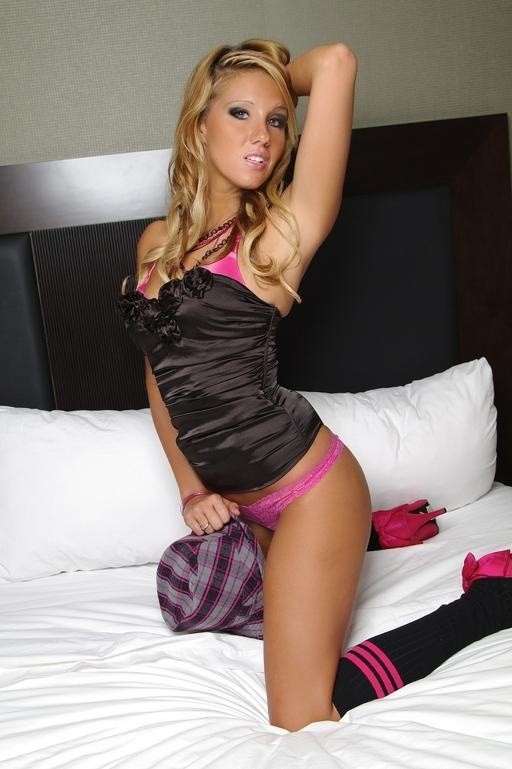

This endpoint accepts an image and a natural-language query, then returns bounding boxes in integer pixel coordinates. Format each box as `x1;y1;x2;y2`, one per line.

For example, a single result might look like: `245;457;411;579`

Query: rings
202;523;209;531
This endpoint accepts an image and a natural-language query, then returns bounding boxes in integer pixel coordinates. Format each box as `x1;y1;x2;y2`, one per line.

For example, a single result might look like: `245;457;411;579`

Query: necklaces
175;216;239;273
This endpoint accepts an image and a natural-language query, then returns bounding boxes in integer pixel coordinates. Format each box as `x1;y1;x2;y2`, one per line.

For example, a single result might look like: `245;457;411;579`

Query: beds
2;114;512;769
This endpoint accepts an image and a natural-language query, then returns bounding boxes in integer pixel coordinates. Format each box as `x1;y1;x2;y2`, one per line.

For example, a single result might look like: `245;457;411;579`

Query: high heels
371;498;447;549
460;546;511;592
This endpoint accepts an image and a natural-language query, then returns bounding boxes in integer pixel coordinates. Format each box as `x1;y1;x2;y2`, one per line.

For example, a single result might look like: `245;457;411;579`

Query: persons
119;38;512;730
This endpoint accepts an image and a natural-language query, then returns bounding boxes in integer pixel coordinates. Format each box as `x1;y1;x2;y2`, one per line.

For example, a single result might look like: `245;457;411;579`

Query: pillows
2;404;191;583
296;358;498;512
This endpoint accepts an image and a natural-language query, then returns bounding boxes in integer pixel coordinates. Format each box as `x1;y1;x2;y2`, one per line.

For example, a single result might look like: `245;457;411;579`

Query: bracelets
180;491;210;510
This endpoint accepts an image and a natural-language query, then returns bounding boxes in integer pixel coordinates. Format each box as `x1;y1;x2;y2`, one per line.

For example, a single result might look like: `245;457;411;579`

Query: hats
157;514;269;642
